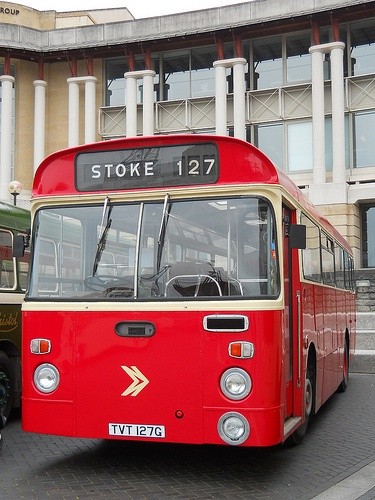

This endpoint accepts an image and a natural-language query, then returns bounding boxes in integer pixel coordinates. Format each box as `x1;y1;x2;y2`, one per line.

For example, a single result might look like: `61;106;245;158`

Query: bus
0;203;138;429
19;135;358;447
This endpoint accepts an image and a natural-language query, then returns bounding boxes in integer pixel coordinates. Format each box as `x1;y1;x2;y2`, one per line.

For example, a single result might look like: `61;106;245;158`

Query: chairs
173;262;237;283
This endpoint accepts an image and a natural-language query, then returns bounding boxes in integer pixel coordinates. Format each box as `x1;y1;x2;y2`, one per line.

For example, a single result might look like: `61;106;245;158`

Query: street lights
7;178;23;204
